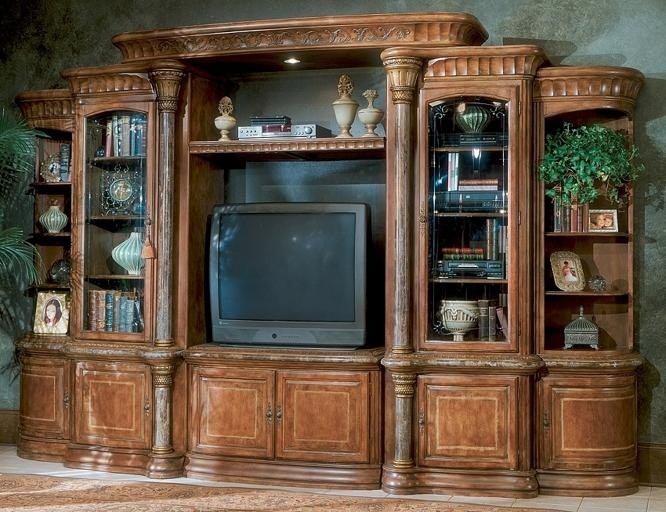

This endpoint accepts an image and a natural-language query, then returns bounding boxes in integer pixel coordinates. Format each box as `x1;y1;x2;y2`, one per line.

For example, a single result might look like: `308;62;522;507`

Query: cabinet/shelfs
16;78;156;454
179;51;385;468
410;53;640;467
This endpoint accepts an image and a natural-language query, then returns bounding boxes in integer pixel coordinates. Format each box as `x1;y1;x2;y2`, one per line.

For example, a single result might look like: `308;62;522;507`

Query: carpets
0;464;577;512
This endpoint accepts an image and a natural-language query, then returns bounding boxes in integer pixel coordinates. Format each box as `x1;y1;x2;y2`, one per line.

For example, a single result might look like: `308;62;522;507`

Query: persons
562;261;577;281
44;299;62;326
590;213;614;230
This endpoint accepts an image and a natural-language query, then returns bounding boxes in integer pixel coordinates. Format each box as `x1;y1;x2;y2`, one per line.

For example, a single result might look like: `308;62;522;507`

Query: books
103;114;147;157
447;152;499;191
440;217;507;262
87;288;144;333
478;292;508;342
553;184;589;234
249;115;292;125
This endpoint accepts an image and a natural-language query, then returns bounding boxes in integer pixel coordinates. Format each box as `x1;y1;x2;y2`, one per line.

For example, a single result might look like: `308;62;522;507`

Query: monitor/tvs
208;202;372;345
